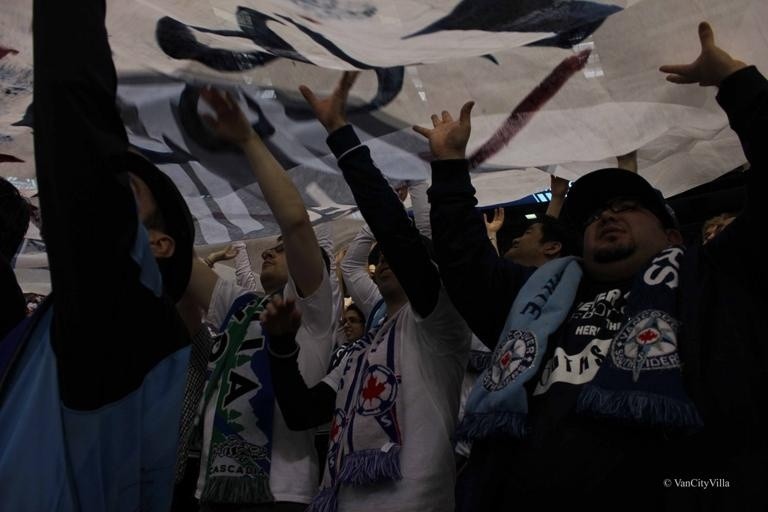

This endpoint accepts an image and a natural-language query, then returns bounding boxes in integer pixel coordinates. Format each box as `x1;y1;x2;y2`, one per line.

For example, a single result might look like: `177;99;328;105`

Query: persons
259;69;474;512
124;83;340;510
410;19;766;511
201;145;741;351
1;0;195;509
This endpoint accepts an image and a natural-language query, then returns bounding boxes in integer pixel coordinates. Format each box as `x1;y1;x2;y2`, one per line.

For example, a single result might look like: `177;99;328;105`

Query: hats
557;168;678;256
